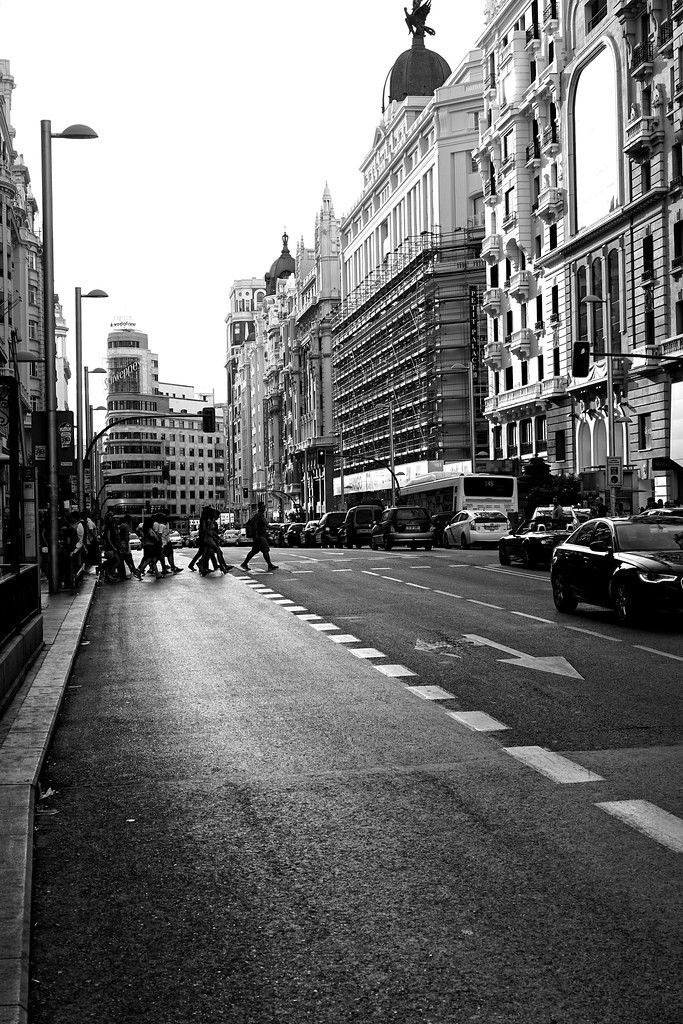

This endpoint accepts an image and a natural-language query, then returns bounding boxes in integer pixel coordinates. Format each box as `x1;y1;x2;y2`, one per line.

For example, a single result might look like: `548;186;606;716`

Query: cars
441;509;512;551
430;511;455;548
498;516;613;568
369;505;437;552
312;511;348;549
338;504;384;549
547;514;683;627
529;506;591;531
636;508;683;517
128;504;318;548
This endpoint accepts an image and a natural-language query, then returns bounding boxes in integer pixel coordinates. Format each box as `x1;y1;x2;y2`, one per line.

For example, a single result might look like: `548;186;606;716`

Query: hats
106;511;114;517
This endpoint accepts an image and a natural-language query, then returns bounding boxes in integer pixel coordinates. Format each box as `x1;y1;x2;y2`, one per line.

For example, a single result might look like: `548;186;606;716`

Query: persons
188;506;234;576
552;496;564;520
135;514;184;582
614;503;626;517
240;502;279;572
40;510;137;592
639;497;683;514
590;496;607;518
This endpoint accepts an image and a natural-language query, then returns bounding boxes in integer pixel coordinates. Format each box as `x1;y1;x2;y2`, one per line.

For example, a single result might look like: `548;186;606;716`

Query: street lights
84;364;107;512
73;287;109;510
89;403;107;503
41;118;97;592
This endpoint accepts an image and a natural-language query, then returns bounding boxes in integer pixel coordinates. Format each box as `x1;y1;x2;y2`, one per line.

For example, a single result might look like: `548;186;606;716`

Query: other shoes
162;570;172;574
241;563;251;570
196;562;234;577
82;576;89;581
171;567;183;572
140;573;145;576
268;564;279;571
95;567;99;575
166;566;170;569
156;575;166;579
103;574;131;583
134;574;143;581
147;569;152;573
151;571;157;575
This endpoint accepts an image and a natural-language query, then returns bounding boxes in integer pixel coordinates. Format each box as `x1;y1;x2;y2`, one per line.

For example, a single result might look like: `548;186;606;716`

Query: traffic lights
317;449;327;465
570;341;590;377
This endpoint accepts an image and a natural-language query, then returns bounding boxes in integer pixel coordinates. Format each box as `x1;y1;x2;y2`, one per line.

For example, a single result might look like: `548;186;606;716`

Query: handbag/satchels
142;539;154;547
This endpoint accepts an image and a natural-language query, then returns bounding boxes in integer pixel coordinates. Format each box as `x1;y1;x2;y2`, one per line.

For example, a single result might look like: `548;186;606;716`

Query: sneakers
188;565;197;571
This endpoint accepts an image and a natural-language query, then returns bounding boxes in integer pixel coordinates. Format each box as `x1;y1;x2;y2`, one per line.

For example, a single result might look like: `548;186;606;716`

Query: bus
390;470;518;531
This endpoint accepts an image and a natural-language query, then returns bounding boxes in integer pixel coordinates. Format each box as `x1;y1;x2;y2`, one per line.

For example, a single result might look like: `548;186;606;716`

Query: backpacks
246;518;256;538
